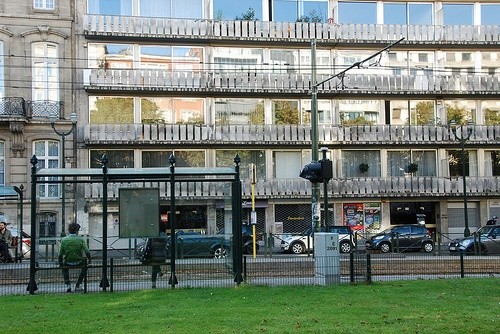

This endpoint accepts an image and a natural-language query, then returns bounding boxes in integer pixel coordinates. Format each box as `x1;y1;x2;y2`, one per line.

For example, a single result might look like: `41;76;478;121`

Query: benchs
35;262;171;293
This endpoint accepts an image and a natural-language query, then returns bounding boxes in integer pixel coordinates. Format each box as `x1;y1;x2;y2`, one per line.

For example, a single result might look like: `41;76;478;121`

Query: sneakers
75;286;83;291
66;285;71;292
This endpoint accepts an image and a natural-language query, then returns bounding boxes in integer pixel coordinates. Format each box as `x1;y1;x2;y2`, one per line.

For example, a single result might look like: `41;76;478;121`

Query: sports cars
133;230;233;261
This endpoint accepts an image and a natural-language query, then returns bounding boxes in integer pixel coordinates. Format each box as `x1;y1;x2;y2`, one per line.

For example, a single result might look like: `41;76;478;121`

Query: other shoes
152;283;156;289
157;271;166;277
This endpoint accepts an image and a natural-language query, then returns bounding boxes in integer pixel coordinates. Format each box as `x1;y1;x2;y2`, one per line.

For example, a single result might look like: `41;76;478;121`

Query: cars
8;228;32;261
242;223;260;254
448;224;500;257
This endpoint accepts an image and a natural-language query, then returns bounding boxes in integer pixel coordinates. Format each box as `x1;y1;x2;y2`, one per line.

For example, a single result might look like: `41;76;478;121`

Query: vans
365;224;434;254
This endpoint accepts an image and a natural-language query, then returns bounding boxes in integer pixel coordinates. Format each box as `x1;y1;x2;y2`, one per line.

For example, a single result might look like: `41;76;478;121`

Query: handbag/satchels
11;236;18;246
141;237;152;266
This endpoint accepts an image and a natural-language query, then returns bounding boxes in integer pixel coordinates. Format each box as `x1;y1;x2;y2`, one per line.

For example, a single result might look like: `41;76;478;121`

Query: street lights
453;128;472;241
298;147;333;232
51;121;77;237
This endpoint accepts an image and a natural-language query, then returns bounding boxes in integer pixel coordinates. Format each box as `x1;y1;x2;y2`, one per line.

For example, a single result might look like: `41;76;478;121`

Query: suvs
280;226;356;254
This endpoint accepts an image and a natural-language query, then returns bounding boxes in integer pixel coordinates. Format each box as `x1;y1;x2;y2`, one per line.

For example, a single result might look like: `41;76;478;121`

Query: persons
0;222;12;262
143;224;167;288
59;223;93;292
486;216;499;225
